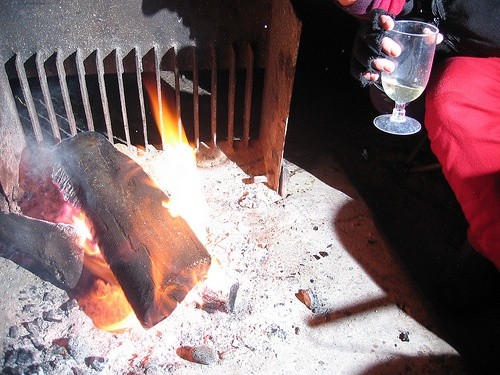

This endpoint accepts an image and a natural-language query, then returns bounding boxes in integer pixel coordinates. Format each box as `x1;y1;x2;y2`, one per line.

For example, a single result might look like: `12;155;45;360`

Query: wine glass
372;21;439;136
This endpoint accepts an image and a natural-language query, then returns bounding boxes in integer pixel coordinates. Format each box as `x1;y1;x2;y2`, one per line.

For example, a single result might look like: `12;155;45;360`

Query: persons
338;0;500;375
